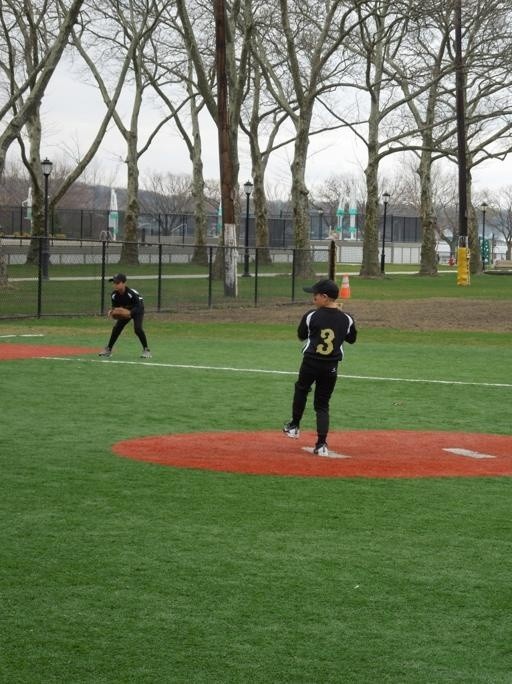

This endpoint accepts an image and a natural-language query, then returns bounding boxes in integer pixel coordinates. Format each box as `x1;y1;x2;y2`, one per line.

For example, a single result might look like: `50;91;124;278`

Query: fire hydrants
448;254;457;266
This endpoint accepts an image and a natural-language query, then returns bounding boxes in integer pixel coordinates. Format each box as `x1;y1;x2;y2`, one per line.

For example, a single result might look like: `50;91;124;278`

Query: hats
303;279;338;299
108;273;127;284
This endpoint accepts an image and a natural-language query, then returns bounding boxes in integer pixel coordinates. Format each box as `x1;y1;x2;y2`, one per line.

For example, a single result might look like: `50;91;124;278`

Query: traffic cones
338;273;351;299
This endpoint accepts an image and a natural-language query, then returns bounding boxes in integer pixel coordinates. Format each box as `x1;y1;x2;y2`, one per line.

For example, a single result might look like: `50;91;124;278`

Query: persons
96;272;153;360
280;278;357;458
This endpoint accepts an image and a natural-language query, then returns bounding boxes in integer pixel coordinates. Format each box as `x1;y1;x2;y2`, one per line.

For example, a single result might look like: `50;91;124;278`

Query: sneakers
283;425;300;439
141;348;151;358
314;445;328;456
99;347;111;356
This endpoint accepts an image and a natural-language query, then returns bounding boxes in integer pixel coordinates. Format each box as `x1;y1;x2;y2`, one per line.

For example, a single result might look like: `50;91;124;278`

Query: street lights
39;156;54;277
379;190;391;272
240;179;256;278
479;202;489;270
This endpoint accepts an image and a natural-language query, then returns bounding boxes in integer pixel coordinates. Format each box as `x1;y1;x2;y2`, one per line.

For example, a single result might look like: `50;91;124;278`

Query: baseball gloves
109;308;130;319
